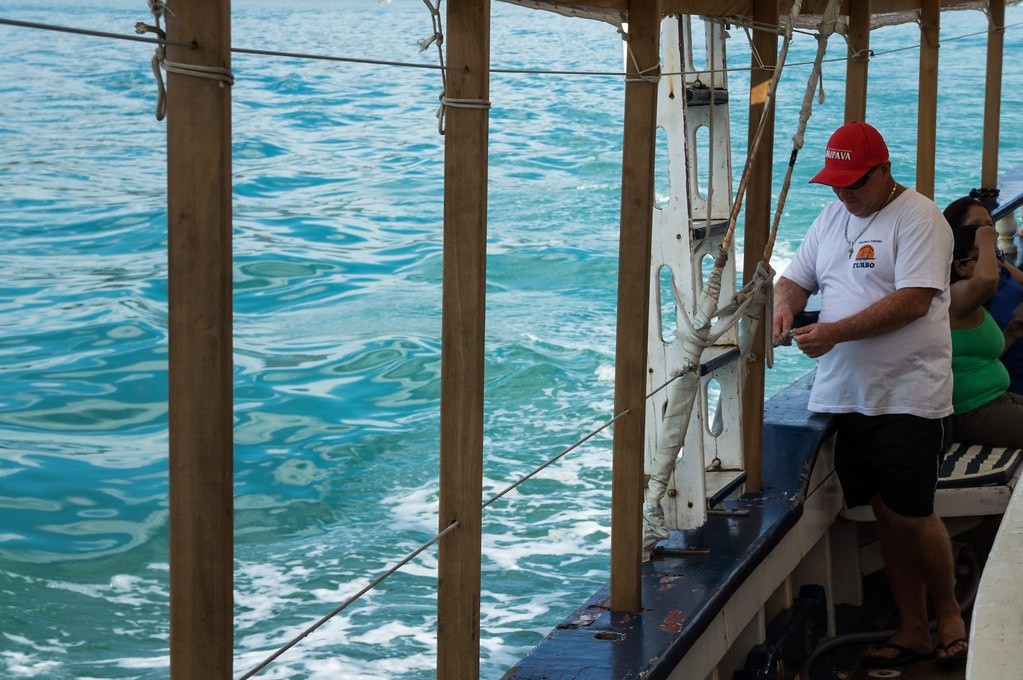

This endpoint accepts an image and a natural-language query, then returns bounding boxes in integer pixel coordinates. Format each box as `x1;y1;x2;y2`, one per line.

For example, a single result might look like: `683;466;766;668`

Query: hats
809;120;889;188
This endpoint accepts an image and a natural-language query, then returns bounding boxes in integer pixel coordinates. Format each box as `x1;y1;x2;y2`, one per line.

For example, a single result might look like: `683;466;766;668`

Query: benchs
838;441;1023;521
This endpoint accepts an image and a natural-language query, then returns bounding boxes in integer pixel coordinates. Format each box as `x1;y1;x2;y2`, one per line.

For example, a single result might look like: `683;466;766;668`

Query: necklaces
845;181;897;259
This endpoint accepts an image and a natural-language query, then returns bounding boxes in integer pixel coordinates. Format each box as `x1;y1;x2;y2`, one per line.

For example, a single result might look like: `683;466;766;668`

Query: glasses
954;250;1004;265
848;166;878;190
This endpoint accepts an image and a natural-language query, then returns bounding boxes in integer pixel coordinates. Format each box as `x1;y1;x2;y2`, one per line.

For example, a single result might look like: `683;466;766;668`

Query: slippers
935;638;968;666
864;643;936;666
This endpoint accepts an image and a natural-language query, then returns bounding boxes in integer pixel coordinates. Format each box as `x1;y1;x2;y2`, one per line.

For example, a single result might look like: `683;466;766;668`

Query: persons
944;196;1023;396
949;226;1023;451
774;121;970;667
